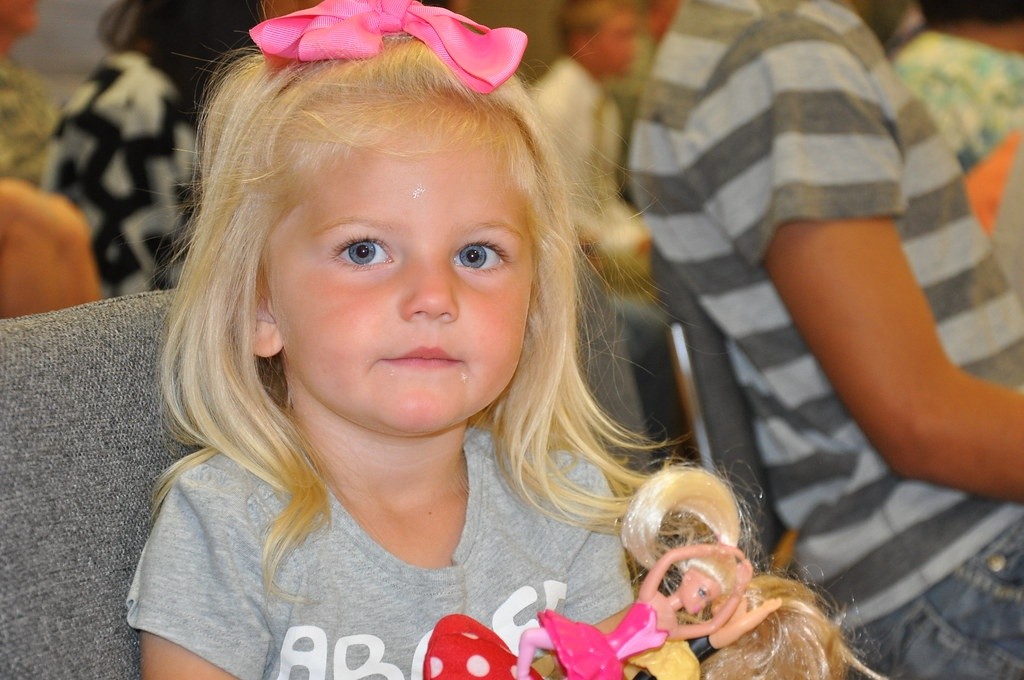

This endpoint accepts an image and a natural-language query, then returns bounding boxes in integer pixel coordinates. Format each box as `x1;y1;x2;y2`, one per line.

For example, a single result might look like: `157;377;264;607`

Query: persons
127;0;664;680
425;466;845;680
0;0;471;321
524;0;1024;680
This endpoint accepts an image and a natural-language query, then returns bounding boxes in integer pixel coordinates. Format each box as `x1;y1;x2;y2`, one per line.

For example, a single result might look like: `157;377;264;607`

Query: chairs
662;276;781;568
0;286;650;680
